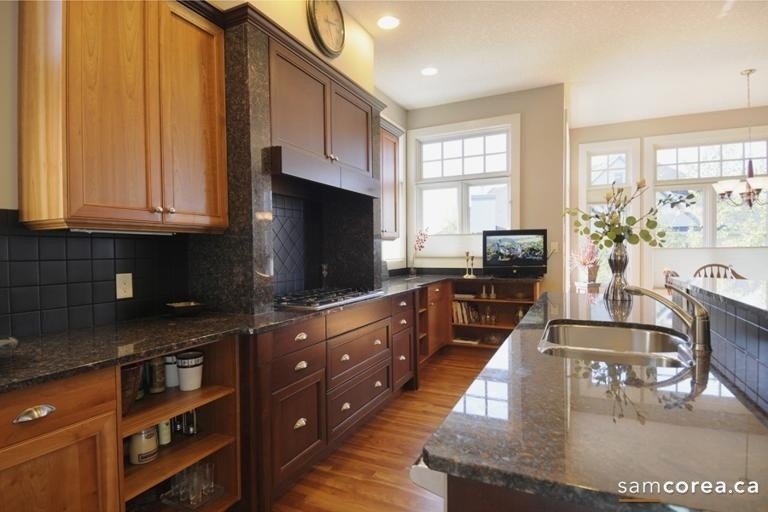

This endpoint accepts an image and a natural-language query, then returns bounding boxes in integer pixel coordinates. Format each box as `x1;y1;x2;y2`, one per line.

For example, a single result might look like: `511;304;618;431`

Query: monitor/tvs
483;228;547;280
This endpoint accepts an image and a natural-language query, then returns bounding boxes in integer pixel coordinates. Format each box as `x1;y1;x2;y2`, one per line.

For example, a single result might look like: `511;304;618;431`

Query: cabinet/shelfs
326;292;390;451
1;328;249;512
387;288;417;406
417;277;449;369
15;1;230;242
267;38;373;173
241;310;327;511
449;277;540;351
379;115;404;241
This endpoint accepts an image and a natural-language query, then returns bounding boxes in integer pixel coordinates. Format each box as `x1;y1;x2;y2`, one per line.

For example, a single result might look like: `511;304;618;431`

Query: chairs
692;262;748;281
662;268;682;295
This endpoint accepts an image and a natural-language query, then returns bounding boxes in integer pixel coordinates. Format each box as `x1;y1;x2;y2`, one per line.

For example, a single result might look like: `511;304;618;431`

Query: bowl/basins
166;300;204;319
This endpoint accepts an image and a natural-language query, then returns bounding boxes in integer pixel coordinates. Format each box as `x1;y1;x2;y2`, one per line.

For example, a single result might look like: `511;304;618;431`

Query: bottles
134;356;180;399
158;405;197;446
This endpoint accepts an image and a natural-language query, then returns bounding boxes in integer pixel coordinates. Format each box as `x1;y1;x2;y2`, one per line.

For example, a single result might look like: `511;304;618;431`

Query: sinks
545;320;686;354
540;346;688;369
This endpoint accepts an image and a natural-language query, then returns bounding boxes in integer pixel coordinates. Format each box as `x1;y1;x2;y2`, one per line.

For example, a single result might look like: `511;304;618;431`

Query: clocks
304;1;351;59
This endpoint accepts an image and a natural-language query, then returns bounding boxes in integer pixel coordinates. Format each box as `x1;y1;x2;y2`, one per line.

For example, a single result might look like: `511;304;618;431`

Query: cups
170;461;216;506
120;363;144;419
177;352;204;394
479;304;498;325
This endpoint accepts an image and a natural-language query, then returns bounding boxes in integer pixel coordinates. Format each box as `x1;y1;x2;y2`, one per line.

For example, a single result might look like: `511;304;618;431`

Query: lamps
709;66;767;208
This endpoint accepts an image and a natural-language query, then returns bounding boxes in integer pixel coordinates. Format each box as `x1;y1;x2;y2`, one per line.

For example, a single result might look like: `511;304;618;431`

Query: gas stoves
272;284;385;312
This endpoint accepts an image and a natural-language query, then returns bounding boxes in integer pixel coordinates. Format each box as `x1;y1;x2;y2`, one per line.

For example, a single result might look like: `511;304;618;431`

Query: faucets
624;284;694;331
623;359;711;411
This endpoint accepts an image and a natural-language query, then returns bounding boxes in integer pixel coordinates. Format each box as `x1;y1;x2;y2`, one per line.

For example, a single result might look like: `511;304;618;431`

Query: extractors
272;145;381;202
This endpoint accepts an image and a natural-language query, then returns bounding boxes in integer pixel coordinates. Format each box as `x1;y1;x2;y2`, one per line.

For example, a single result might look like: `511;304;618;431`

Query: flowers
561;176;699;260
563;355;698;427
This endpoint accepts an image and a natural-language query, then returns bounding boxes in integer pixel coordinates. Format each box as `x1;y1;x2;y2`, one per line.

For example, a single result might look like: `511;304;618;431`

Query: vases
604;300;634;322
577;263;600;283
601;235;631;300
